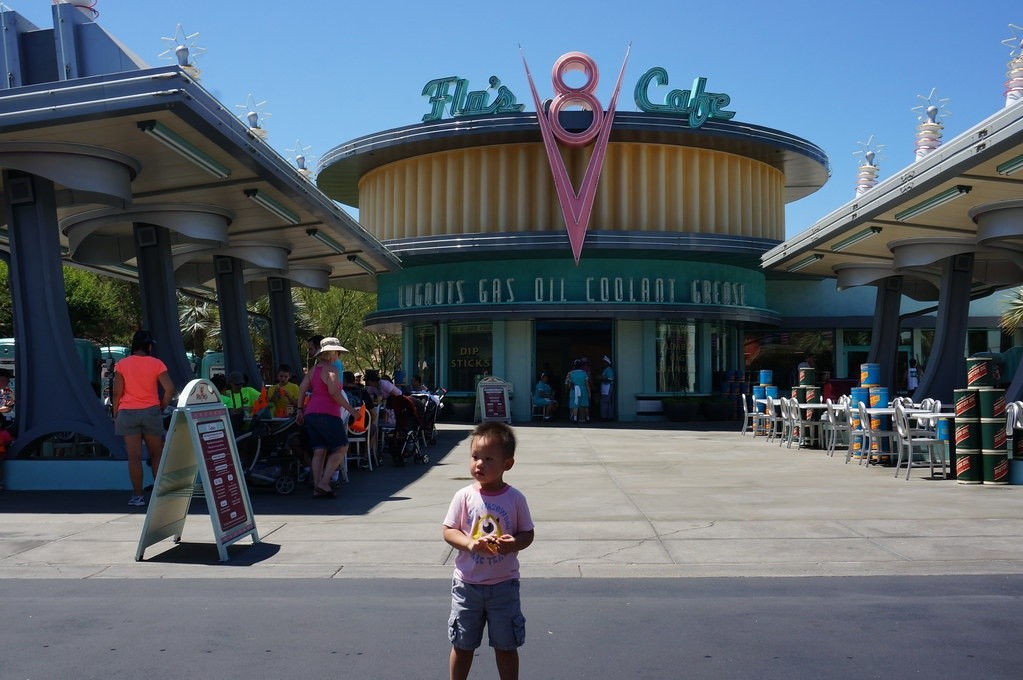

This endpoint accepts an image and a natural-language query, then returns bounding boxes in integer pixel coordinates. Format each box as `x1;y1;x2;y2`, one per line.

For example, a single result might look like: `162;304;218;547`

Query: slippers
315;486;339;498
314;491;325;499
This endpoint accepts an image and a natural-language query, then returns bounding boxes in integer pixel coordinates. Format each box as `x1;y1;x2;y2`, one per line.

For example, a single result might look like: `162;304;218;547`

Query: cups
286;405;293;417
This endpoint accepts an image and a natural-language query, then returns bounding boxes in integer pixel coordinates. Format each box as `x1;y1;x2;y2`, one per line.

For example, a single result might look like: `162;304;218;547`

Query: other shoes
127;494;146;506
585;420;591;424
573;420;578;425
544;417;552;422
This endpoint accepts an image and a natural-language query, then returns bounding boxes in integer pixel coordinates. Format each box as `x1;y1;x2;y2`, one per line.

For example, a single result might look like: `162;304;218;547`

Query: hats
313;337;351;356
228;371;247;385
0;368;15;378
363;372;381;382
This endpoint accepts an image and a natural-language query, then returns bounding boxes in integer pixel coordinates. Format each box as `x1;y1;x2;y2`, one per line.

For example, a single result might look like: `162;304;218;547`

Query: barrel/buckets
953;358;1009;485
851;363;893;459
792;368;822;445
938;419;949;444
753;370;779;436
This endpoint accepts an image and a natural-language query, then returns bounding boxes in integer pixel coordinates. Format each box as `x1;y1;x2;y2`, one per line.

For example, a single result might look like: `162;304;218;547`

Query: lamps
995;153;1023;176
349;255;376;275
787;254;824;272
894;185;972;223
243;188;301;225
831;227;882;252
305;228;345;255
136;119;231;180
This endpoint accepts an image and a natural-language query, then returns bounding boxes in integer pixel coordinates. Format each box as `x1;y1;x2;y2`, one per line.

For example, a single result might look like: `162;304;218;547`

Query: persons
210;371;262;460
307;335;349;480
444;421;535;680
0;370;15;426
596;356;614;419
532;372;558;420
264;365;301;417
342;367;429;468
112;330;174;506
296;337;361;500
903;357;925;395
565;356;594;422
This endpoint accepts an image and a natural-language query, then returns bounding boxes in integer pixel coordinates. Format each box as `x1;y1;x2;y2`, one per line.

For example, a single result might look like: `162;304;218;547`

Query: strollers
421;386;448;447
377;392;430;468
235;406;305;496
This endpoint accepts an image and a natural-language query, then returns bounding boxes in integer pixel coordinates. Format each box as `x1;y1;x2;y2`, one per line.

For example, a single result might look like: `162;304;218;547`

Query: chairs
530;393;546;422
741;393;945;482
345;405;394;472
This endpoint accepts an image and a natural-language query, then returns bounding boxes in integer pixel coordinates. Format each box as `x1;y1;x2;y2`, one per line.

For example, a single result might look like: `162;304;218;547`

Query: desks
755;398;790;438
790;402;846;449
912;413;957;480
848;407;932;467
242;417;293;464
